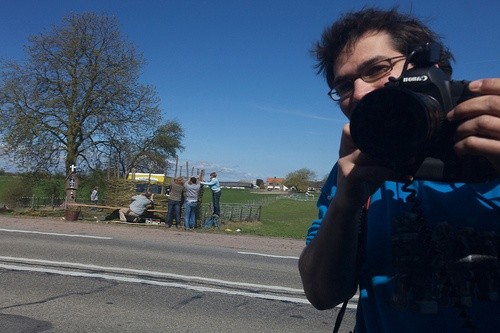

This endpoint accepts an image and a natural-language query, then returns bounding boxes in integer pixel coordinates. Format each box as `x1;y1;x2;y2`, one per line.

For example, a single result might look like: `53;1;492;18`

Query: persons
298;3;500;333
201;172;221;218
165;177;185;228
119;192;154;222
184;177;201;229
90;186;98;205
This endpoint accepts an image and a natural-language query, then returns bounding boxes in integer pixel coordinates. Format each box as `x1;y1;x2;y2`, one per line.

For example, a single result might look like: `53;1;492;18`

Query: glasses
327;54;406;101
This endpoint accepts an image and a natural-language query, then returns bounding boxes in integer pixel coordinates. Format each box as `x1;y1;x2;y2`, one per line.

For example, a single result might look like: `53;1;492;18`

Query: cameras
350;40;483;176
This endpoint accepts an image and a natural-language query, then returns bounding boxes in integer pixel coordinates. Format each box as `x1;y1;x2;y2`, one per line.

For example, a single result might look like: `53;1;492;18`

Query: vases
66;209;80;221
